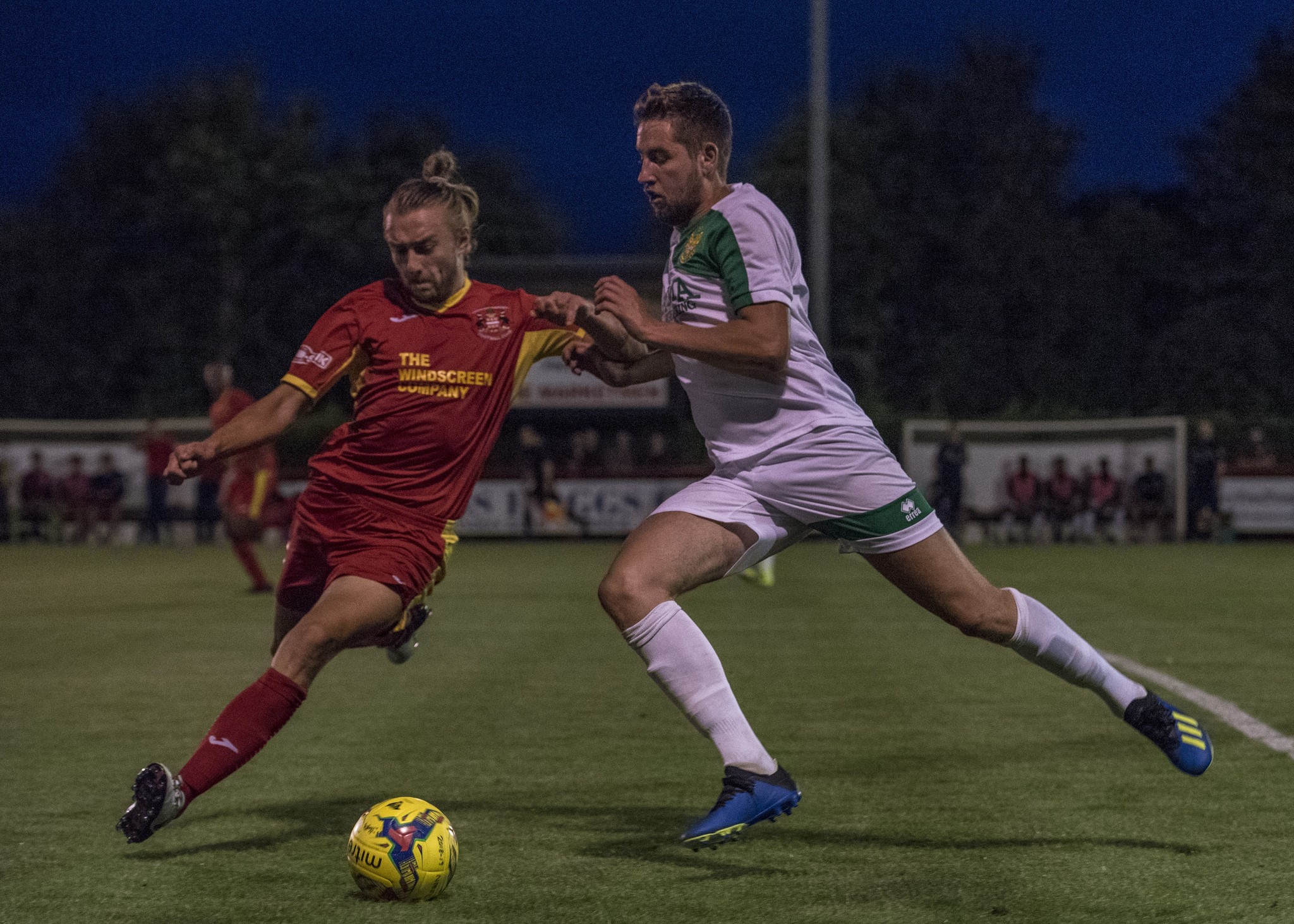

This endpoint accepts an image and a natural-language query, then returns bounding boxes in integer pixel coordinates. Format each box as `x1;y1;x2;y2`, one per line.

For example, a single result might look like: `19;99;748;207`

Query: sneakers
386;604;433;665
1122;687;1216;776
679;762;802;852
116;762;185;843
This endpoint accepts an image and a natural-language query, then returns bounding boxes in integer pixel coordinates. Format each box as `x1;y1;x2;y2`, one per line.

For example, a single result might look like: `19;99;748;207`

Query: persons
0;451;132;546
201;358;300;593
561;80;1214;853
1002;449;1177;544
134;417;339;547
1189;417;1276;543
931;419;971;548
114;148;648;846
476;408;716;481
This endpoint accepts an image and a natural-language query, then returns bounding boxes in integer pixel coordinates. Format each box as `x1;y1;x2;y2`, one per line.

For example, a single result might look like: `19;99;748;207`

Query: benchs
18;508;223;546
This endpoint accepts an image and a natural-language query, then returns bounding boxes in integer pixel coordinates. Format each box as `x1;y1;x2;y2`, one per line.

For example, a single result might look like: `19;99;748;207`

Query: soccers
345;795;461;904
